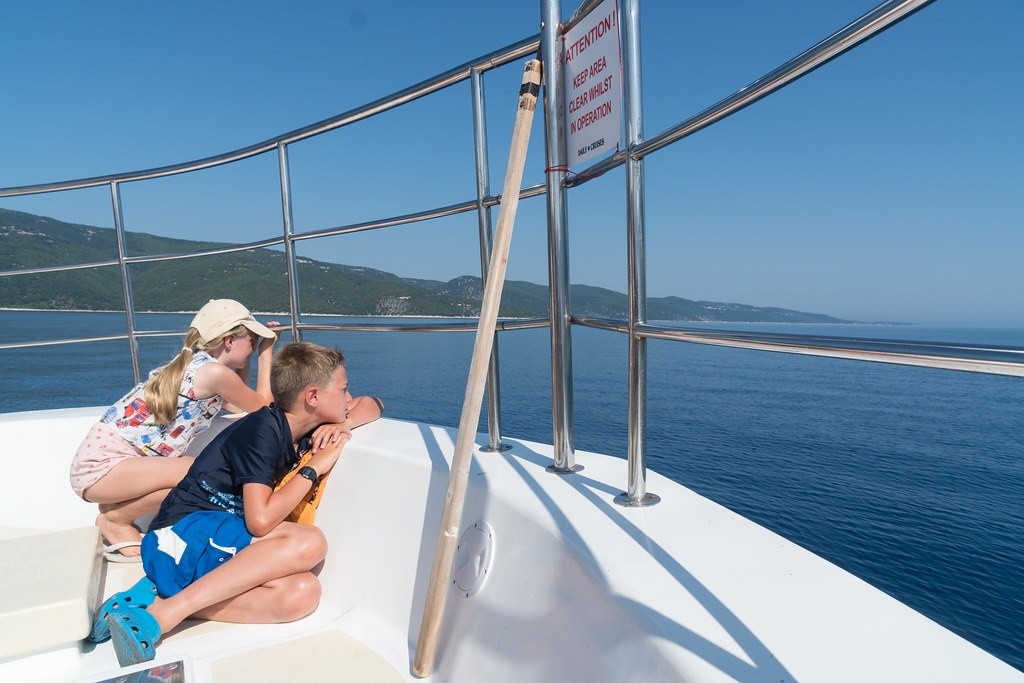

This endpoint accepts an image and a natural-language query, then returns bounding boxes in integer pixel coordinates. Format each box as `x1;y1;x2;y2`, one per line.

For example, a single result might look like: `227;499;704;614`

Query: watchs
296;466;320;487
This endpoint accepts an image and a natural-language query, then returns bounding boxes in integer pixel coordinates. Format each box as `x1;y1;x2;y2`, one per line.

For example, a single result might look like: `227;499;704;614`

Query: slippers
102;541;142;562
85;576;159;641
107;605;162;667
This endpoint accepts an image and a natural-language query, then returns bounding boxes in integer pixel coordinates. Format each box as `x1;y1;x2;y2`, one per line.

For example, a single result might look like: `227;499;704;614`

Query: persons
86;340;383;669
70;299;283;564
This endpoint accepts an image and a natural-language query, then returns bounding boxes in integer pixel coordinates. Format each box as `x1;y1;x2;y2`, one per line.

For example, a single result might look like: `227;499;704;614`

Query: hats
189;299;276;349
